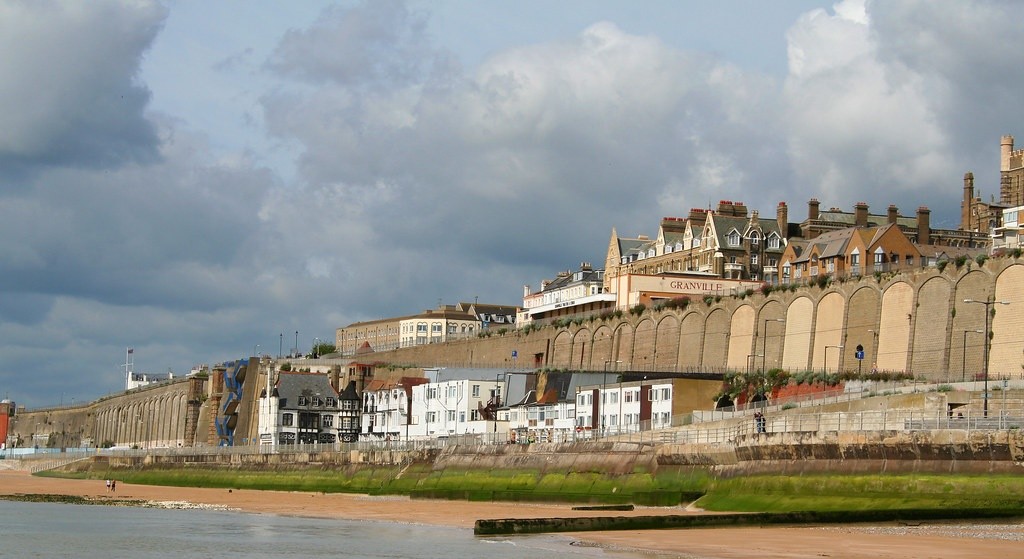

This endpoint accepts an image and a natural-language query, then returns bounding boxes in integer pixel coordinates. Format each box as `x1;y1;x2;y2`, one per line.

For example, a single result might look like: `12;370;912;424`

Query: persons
107;478;116;492
753;412;769;432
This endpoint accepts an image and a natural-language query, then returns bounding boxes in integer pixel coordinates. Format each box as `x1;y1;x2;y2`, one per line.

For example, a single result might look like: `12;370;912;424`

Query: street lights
745;354;763;405
494;373;513;432
963;295;1010;420
761;318;785;412
279;333;283;358
962;329;983;383
295;330;298;358
313;337;318;360
601;361;623;436
823;345;845;391
341;329;344;359
254;345;260;357
